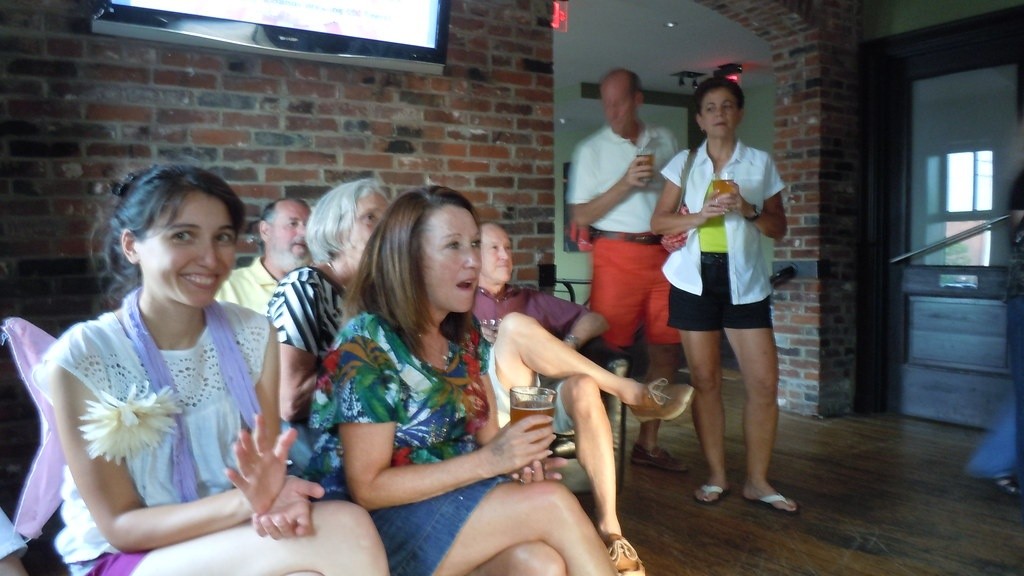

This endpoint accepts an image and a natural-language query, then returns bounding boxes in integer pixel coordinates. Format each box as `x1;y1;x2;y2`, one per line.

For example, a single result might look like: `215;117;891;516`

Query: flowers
74;376;184;467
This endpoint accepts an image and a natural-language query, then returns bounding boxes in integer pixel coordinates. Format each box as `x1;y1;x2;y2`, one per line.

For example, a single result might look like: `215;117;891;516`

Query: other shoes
632;442;690;471
982;474;1024;496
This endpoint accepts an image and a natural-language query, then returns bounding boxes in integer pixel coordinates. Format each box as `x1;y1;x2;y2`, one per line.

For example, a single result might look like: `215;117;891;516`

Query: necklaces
328;263;348;292
419;334;448;361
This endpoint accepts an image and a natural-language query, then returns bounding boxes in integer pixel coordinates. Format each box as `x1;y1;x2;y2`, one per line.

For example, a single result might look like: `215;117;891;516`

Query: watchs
745;204;761;221
565;334;580;350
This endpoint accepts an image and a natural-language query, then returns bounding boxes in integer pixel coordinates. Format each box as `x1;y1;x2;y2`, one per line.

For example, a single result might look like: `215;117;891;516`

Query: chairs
535;336;628;500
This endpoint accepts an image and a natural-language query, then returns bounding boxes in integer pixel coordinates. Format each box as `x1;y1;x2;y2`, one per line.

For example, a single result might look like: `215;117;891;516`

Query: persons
565;67;687;472
0;160;619;576
966;172;1024;495
650;76;800;517
470;222;694;576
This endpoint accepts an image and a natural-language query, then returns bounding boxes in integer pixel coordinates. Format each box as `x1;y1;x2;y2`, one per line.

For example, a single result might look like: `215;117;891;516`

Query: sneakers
603;535;645;576
629;378;696;424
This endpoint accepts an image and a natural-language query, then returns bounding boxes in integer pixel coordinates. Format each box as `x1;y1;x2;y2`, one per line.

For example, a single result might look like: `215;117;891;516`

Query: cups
509;386;557;475
711;173;735;213
635;151;655;182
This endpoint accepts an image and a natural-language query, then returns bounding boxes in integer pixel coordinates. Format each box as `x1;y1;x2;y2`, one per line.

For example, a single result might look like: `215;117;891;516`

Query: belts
701;252;728;265
592;229;663;245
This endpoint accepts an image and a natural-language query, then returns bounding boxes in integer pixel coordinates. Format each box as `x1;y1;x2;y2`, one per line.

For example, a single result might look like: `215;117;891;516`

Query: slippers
693;484;730;506
743;492;800;516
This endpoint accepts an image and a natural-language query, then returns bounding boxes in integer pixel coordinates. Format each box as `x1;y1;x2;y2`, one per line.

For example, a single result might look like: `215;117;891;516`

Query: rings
518;474;524;484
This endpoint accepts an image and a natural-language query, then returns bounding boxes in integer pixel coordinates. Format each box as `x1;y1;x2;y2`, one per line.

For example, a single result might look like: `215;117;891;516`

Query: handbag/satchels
661;148;698;253
